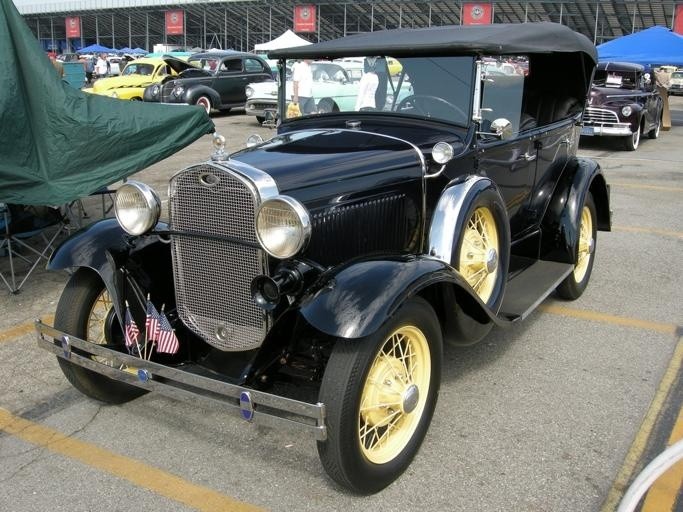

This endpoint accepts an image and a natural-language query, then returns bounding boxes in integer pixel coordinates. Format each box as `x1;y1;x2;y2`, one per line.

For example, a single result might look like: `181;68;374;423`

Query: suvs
35;21;613;498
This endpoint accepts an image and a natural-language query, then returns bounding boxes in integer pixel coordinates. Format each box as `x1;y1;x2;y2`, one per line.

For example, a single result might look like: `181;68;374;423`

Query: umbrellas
76;44;148;55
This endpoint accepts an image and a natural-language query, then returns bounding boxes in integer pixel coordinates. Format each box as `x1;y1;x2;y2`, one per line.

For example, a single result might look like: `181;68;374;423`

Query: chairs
483;90;578;142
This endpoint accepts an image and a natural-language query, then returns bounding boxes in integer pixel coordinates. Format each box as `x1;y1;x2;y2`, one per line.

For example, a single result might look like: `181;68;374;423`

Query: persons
290;58;316;116
354;54;388;111
73;54;133;89
46;49;56;60
656;68;672;131
